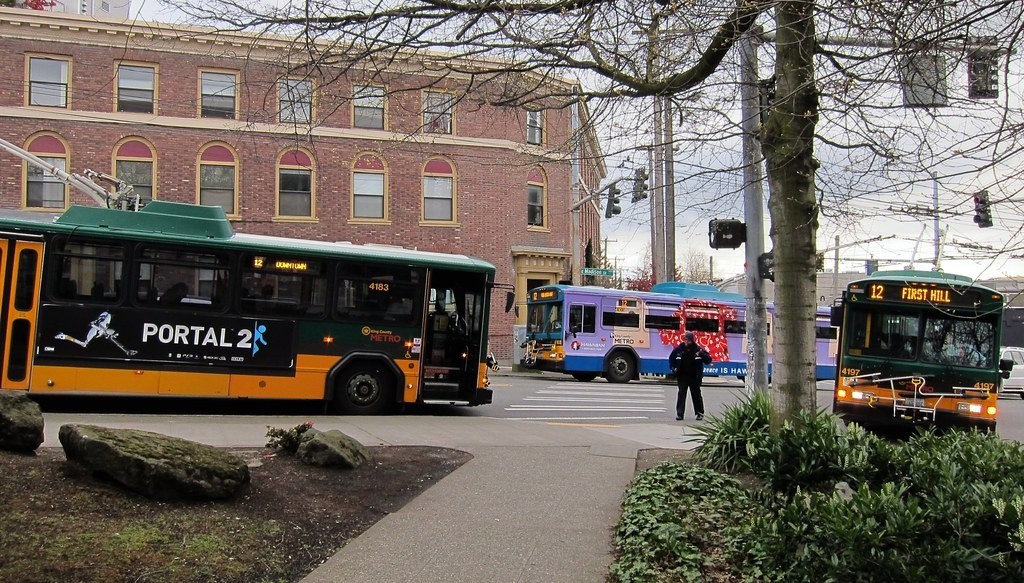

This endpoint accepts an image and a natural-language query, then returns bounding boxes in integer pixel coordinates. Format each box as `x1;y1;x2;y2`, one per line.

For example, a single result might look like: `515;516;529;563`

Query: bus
999;306;1024;400
514;282;841;385
0;197;516;414
828;269;1014;435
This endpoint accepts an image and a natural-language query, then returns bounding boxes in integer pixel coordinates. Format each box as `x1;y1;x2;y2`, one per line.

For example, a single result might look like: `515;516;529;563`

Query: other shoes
696;414;703;420
676;415;682;420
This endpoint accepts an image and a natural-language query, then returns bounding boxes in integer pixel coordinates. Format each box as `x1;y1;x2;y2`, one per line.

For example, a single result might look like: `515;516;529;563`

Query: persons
668;332;712;421
159;281;449;326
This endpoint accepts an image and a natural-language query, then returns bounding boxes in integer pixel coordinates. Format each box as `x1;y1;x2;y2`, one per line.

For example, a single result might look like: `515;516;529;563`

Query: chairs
68;279;187;305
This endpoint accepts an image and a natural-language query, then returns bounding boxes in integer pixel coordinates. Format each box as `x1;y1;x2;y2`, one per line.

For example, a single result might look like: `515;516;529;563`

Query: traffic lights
631;166;649;203
972;191;994;228
605;184;622;218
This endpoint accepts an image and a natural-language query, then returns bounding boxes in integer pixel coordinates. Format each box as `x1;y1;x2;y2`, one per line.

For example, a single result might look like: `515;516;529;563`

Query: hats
685;330;694;342
